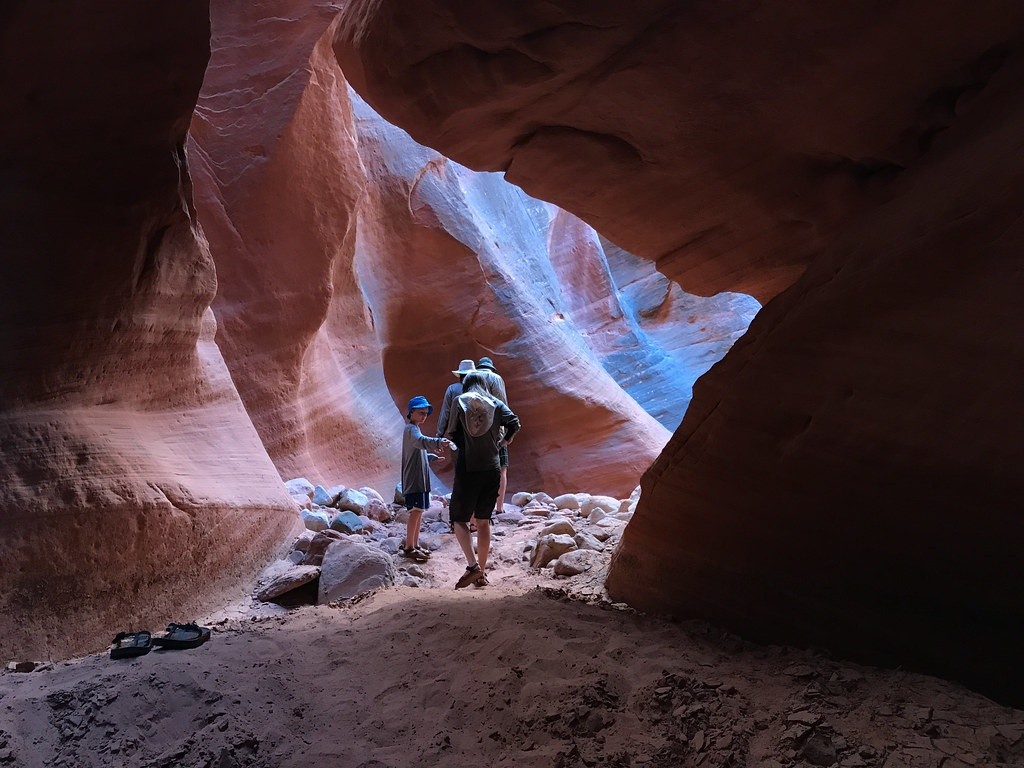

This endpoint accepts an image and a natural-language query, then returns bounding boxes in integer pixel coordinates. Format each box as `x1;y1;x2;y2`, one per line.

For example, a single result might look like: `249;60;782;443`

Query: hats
475;356;496;372
452;360;479;378
406;397;433;420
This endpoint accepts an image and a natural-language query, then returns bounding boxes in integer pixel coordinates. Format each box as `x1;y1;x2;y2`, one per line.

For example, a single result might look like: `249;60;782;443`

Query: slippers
154;620;210;647
110;631;153;658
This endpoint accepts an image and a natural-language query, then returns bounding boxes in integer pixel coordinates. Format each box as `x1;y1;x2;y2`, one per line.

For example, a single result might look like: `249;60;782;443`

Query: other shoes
496;510;505;515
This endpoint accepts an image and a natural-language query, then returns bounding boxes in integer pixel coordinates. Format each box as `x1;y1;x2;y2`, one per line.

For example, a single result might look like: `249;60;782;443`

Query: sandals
399;547;430;559
470;523;477;533
456;563;483;588
475;572;489;587
450;523;456;533
415;546;431;555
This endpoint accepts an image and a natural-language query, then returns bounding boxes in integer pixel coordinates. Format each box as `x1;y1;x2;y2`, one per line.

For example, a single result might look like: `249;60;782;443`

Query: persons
402;396;451;559
435;360;477;453
476;356;509;515
448;370;522;590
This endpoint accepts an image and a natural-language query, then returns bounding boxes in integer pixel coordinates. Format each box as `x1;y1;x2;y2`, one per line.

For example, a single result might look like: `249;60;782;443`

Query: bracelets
498;441;502;448
